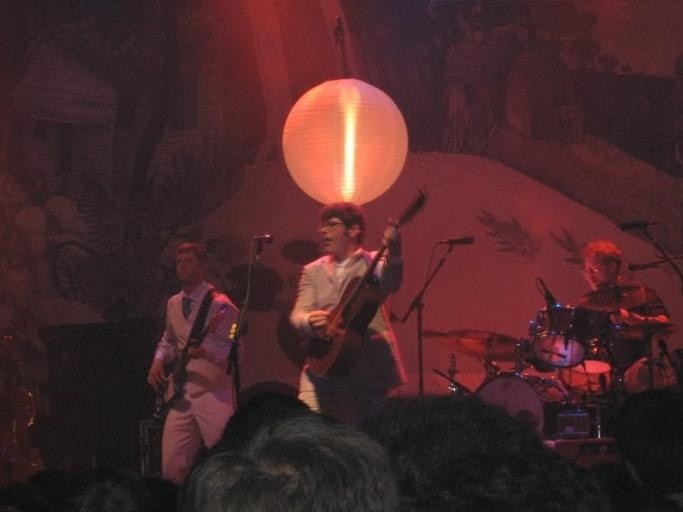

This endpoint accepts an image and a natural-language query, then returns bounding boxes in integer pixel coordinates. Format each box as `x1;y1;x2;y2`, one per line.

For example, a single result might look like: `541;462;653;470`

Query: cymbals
446;330;532;362
577;286;657;311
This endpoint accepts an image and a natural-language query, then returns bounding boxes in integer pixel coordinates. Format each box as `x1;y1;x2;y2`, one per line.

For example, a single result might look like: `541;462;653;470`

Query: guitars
154;338;199;418
305;190;427;383
0;326;46;484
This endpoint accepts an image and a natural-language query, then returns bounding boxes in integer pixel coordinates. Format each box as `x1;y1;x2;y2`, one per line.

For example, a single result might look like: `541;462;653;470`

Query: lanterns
282;77;411;208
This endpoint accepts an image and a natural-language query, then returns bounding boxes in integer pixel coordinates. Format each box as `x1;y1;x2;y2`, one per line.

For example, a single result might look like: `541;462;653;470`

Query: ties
183;297;192;318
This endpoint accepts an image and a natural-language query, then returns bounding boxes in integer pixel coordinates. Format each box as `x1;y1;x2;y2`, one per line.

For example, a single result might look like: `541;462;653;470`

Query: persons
287;204;405;428
148;241;243;483
579;241;672;386
1;381;682;511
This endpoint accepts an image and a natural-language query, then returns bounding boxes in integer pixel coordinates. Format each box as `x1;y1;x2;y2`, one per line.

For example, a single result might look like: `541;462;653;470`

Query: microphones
619;218;648;232
253;233;276;247
445;234;474;246
627;261;656;273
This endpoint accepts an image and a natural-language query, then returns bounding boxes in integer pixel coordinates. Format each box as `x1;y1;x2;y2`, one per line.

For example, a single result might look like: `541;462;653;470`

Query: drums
474;372;577;441
575;327;615;375
533;304;588;368
